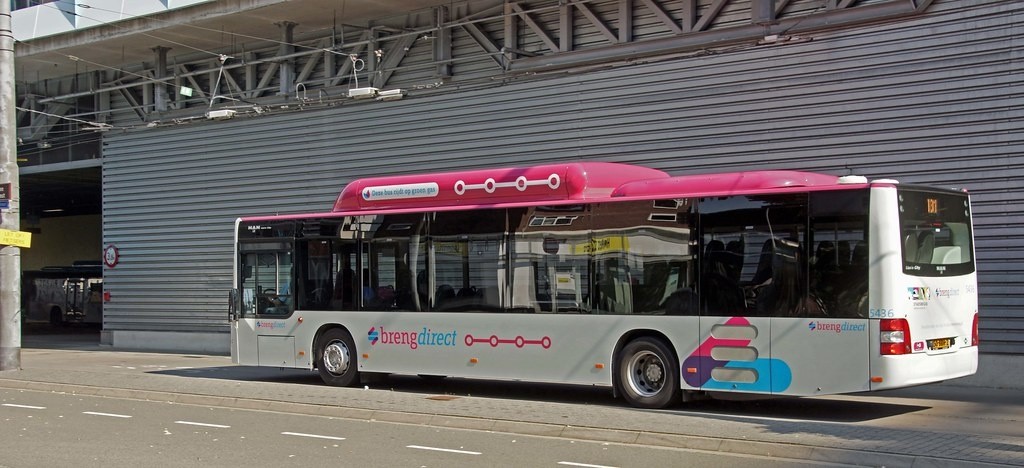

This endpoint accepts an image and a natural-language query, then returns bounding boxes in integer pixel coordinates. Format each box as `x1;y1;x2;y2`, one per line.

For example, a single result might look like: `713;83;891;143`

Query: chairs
704;239;868;317
365;284;499;308
904;231;935;263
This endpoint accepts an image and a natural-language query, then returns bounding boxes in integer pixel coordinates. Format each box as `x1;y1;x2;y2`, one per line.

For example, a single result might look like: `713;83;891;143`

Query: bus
23;268;103;329
227;135;981;410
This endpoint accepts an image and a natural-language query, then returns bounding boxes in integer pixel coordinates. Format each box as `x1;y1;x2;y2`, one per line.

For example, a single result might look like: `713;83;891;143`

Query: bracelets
271;298;274;303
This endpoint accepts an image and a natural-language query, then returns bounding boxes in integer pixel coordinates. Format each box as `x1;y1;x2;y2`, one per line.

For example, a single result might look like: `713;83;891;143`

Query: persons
593;238;824;317
267;267;296;312
